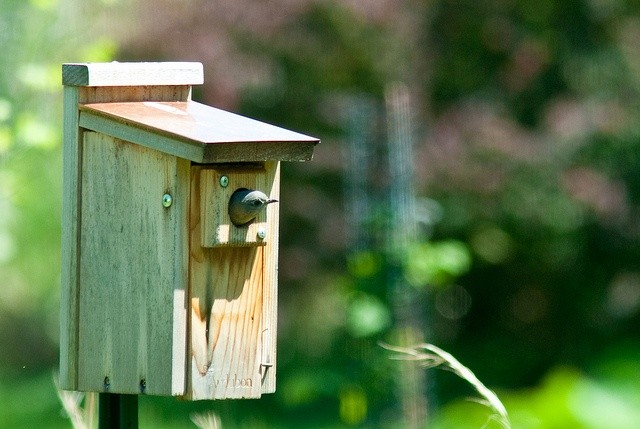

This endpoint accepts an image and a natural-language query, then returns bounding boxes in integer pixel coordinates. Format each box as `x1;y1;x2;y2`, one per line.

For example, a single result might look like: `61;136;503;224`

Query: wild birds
230;189;279;224
